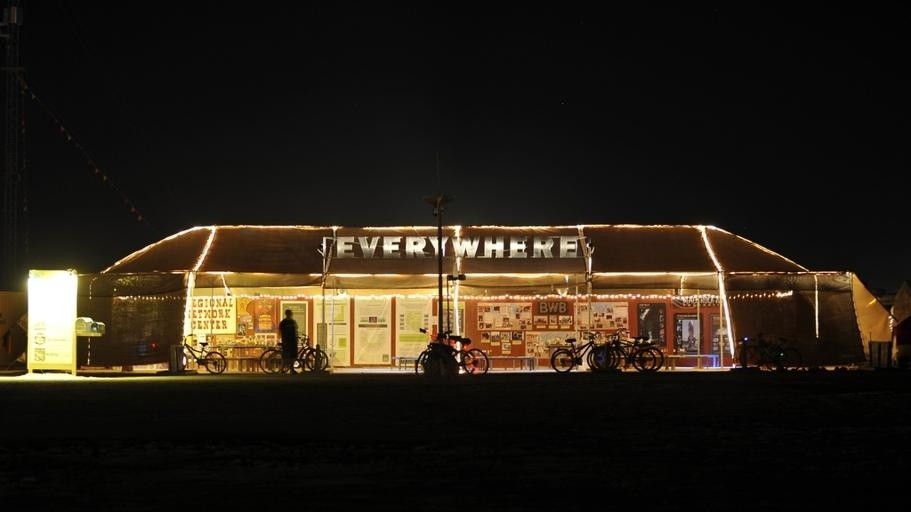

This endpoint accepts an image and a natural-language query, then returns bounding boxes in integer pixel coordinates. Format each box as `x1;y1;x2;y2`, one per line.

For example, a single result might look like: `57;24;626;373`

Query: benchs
203;355;313;373
608;353;721;369
392;353;540;372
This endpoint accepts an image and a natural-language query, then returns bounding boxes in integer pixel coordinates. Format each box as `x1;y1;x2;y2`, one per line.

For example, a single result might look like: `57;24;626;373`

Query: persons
6;313;27;359
281;310;298;375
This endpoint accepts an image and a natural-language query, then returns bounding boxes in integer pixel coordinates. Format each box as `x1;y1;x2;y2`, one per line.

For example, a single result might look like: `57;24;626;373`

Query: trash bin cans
170;345;183;372
870;341;891;368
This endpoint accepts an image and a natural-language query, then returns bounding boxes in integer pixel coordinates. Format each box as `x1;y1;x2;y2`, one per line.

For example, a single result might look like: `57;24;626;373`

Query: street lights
445;273;466;345
422;191;457;346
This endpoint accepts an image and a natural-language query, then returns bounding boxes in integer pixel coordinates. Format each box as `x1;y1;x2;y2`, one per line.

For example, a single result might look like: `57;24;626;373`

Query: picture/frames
637;302;731;355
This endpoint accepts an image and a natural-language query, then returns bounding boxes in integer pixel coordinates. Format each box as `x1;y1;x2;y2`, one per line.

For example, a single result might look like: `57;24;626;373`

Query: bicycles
258;334;328;374
549;327;664;373
414;327;491;375
171;334;227;375
732;338;810;370
